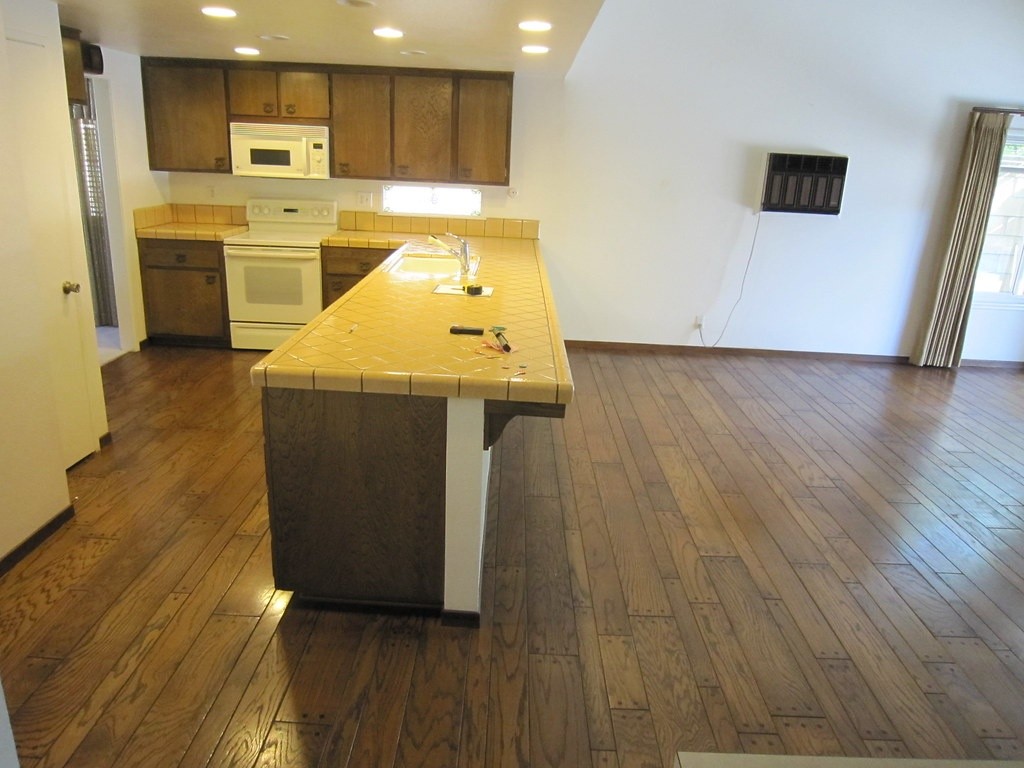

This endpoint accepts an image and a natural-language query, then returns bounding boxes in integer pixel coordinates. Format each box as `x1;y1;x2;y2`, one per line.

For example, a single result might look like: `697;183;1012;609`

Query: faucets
427;231;470;274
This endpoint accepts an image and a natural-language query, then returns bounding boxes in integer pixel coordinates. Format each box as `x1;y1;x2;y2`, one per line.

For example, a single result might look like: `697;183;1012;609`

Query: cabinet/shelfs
138;236;229;350
322;248;395;309
143;63;512;184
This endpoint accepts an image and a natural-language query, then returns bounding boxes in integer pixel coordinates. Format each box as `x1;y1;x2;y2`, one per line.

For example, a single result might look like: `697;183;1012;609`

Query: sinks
384;252;482;276
